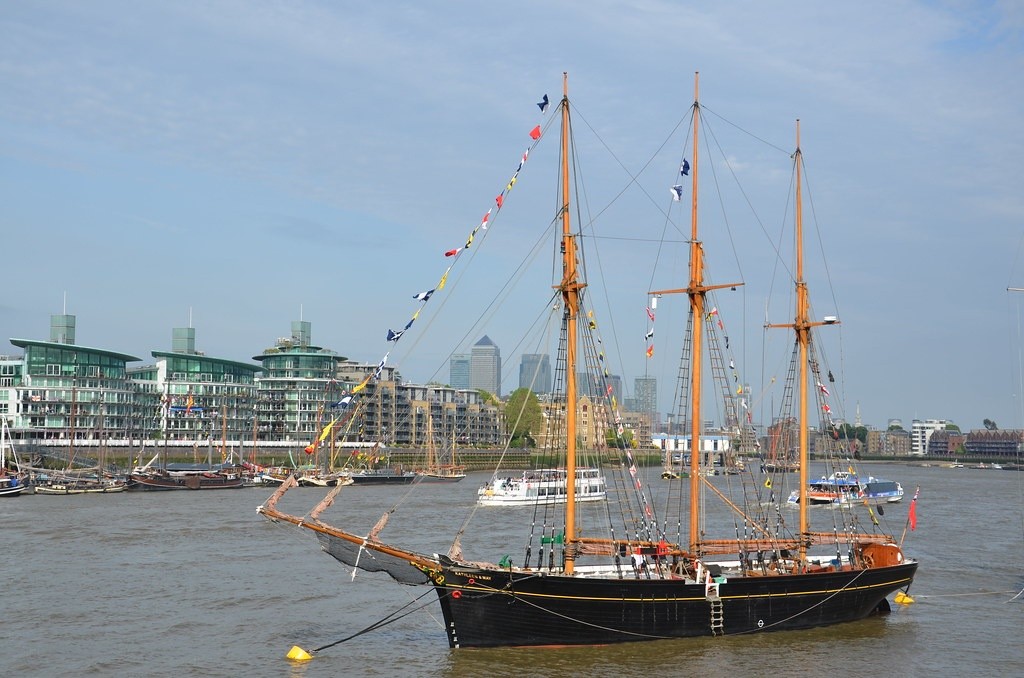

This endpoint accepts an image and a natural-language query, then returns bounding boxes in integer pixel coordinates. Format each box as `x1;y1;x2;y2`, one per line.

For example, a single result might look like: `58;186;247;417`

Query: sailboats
255;69;920;653
0;353;466;498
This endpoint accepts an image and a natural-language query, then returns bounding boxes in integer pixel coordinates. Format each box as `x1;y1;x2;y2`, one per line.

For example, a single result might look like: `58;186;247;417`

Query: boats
758;461;799;474
786;472;905;507
476;467;607;506
661;453;747;480
921;459;1024;472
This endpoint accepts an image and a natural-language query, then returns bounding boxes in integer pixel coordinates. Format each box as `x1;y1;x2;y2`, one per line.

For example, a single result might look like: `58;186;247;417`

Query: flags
588;310;608;378
864;499;878;525
644;308;654;358
709;307;729;349
729;359;748;410
908;498;916;530
373;272;447;380
605;385;636;477
671;159;690;201
445;145;530;257
303;376;369;455
765;478;779;515
748;415;768;474
529;94;549;140
636;479;651;518
839;443;864;498
818;384;838;438
